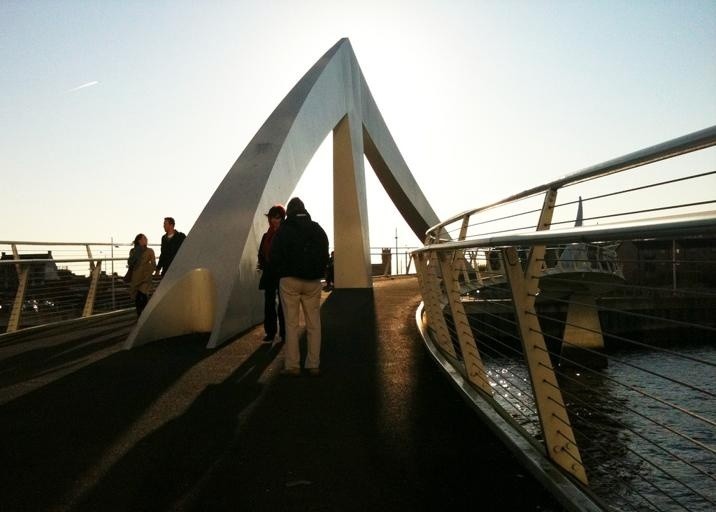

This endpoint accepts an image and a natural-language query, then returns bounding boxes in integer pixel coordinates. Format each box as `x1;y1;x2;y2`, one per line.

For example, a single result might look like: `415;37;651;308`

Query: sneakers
285;366;323;376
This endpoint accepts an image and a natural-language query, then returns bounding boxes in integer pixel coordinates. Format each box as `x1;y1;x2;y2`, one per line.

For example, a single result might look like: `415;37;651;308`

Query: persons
156;217;186;278
123;234;156;315
327;251;334;287
256;203;286;341
269;197;329;379
